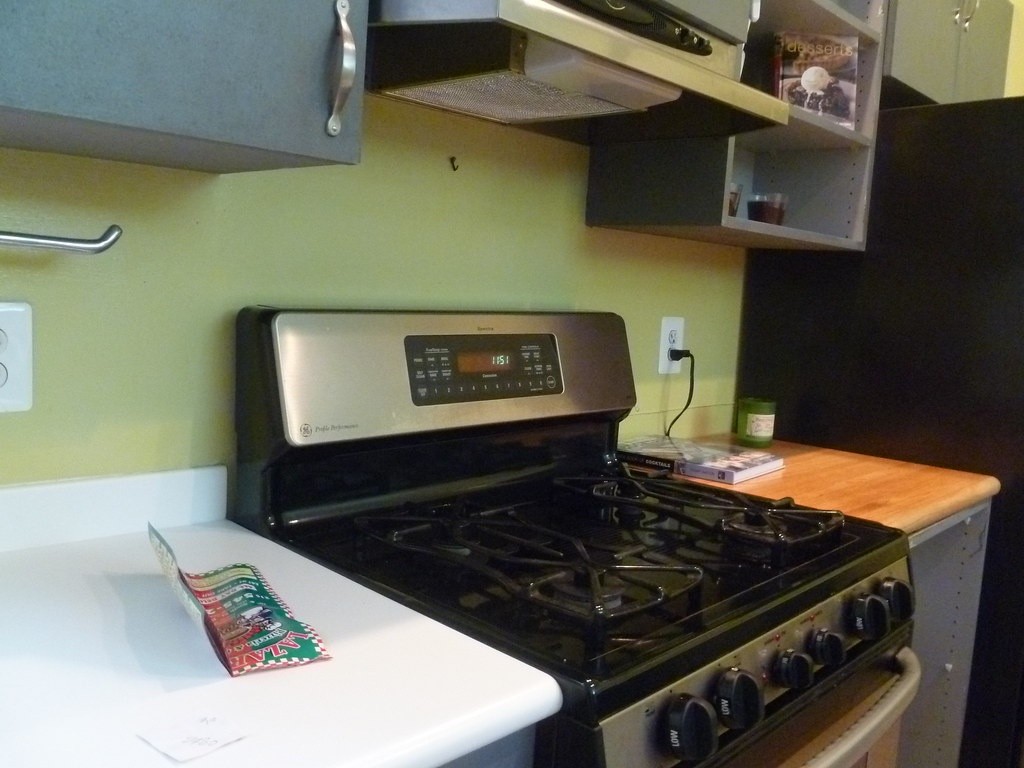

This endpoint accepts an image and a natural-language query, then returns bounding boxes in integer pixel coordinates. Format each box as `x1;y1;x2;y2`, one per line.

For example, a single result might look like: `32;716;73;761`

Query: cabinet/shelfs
585;0;890;251
1;0;369;174
880;0;1014;110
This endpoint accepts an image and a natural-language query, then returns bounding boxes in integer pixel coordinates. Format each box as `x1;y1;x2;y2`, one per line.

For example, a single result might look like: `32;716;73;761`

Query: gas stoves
237;304;914;768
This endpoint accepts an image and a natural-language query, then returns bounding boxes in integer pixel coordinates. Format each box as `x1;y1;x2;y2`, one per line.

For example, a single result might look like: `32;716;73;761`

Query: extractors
365;27;683;126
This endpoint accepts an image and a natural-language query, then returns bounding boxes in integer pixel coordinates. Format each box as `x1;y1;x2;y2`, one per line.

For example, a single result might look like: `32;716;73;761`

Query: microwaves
368;1;762;79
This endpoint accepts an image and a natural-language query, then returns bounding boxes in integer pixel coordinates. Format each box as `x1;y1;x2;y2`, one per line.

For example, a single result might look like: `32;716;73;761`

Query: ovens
713;644;922;766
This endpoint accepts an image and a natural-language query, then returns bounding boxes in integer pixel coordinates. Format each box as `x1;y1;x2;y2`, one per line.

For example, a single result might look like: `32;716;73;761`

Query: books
617;435;786;484
776;30;859;131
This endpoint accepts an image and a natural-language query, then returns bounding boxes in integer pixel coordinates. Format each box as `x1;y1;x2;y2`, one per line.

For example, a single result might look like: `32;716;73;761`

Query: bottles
737;397;777;447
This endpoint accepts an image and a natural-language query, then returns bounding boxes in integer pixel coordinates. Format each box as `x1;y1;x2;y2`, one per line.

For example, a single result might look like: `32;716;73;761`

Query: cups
728;183;744;218
746;194;767;222
767;192;787;224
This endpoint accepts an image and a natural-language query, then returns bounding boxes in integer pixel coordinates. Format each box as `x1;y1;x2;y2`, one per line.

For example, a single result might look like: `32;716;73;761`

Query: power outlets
659;317;685;374
